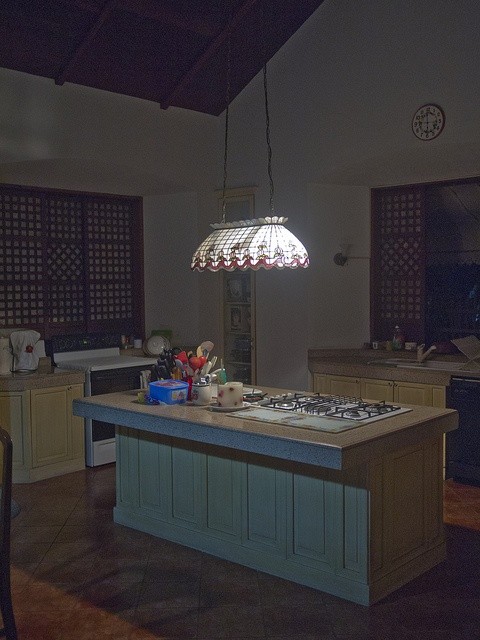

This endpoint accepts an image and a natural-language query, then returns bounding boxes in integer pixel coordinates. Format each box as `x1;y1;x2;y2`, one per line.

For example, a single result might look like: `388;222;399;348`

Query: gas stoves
259;391;412;424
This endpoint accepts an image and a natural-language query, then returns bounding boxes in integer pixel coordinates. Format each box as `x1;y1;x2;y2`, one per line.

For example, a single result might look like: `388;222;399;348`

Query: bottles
392;325;404;350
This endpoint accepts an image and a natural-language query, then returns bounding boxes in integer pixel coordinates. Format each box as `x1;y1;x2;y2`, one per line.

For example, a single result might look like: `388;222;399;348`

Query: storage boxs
149;379;189;404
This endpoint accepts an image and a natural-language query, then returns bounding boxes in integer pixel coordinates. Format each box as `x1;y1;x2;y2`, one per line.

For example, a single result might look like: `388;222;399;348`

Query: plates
242;387;262;394
209;402;251;412
142;335;171;356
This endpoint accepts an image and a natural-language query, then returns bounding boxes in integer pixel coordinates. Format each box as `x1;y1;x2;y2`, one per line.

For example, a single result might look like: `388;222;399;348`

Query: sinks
369;359;464;370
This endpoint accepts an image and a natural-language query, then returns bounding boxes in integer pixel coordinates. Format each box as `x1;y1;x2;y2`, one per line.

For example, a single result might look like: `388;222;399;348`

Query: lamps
190;0;310;272
334;243;353;266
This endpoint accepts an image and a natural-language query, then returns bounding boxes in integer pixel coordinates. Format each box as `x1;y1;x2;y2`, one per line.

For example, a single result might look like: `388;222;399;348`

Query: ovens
54;348;159;466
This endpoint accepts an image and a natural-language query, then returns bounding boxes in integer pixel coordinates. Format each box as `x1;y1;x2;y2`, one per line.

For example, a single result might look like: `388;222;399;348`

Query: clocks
412;104;445;141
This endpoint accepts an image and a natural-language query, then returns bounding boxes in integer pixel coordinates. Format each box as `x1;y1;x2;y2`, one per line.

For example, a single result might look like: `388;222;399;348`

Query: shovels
211;356;218;368
201;341;214;357
187;351;194;358
197;346;209;360
190;356;201;375
175;360;186;378
177;351;189;365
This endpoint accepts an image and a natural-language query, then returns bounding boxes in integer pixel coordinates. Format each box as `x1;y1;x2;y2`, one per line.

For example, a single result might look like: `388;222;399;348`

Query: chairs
0;427;18;640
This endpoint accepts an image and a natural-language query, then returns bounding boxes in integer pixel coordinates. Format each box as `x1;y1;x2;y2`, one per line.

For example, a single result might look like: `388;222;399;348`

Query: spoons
189;356;200;376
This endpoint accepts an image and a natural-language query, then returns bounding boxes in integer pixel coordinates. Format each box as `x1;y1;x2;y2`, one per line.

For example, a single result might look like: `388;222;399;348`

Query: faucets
416;344;438;363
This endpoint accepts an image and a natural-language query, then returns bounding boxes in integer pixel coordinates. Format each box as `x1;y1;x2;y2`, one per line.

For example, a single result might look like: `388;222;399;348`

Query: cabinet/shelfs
0;389;30;485
312;373;331;394
31;384;86;485
217;194;258;387
331;375;393;402
393;380;447;479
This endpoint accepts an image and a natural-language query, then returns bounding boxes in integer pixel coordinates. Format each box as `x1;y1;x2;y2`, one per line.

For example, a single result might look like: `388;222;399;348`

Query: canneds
392;324;406;350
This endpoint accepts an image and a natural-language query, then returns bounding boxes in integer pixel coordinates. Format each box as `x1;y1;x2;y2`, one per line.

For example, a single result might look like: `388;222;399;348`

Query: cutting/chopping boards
151;329;172;340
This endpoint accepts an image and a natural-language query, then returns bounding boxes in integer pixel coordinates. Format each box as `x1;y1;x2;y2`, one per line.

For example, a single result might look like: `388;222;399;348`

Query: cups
134;340;142;348
191;384;212;405
405;342;418;351
